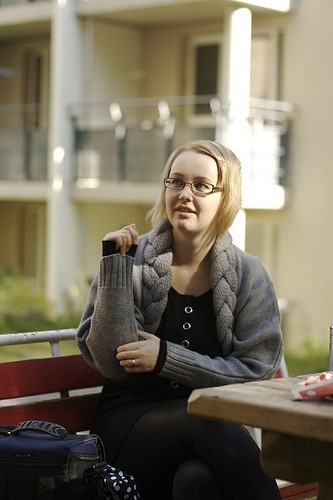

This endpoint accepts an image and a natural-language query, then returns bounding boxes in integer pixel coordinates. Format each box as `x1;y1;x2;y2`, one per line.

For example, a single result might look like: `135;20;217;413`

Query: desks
187;368;333;482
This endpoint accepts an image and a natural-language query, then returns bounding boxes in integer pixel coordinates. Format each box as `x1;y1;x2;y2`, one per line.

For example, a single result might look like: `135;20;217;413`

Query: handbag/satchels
0;420;107;500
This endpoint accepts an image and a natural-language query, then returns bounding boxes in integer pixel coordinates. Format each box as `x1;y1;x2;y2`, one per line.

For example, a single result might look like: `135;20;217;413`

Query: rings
132;360;136;366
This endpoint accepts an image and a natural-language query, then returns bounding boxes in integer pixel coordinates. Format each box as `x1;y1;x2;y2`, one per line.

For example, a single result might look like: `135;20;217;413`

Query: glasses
163;178;221;194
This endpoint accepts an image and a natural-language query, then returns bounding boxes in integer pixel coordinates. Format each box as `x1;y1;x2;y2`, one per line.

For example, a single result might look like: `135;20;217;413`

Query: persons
76;139;285;500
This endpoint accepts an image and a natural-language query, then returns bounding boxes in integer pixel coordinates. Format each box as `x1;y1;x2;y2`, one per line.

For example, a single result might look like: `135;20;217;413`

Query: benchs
0;327;323;500
